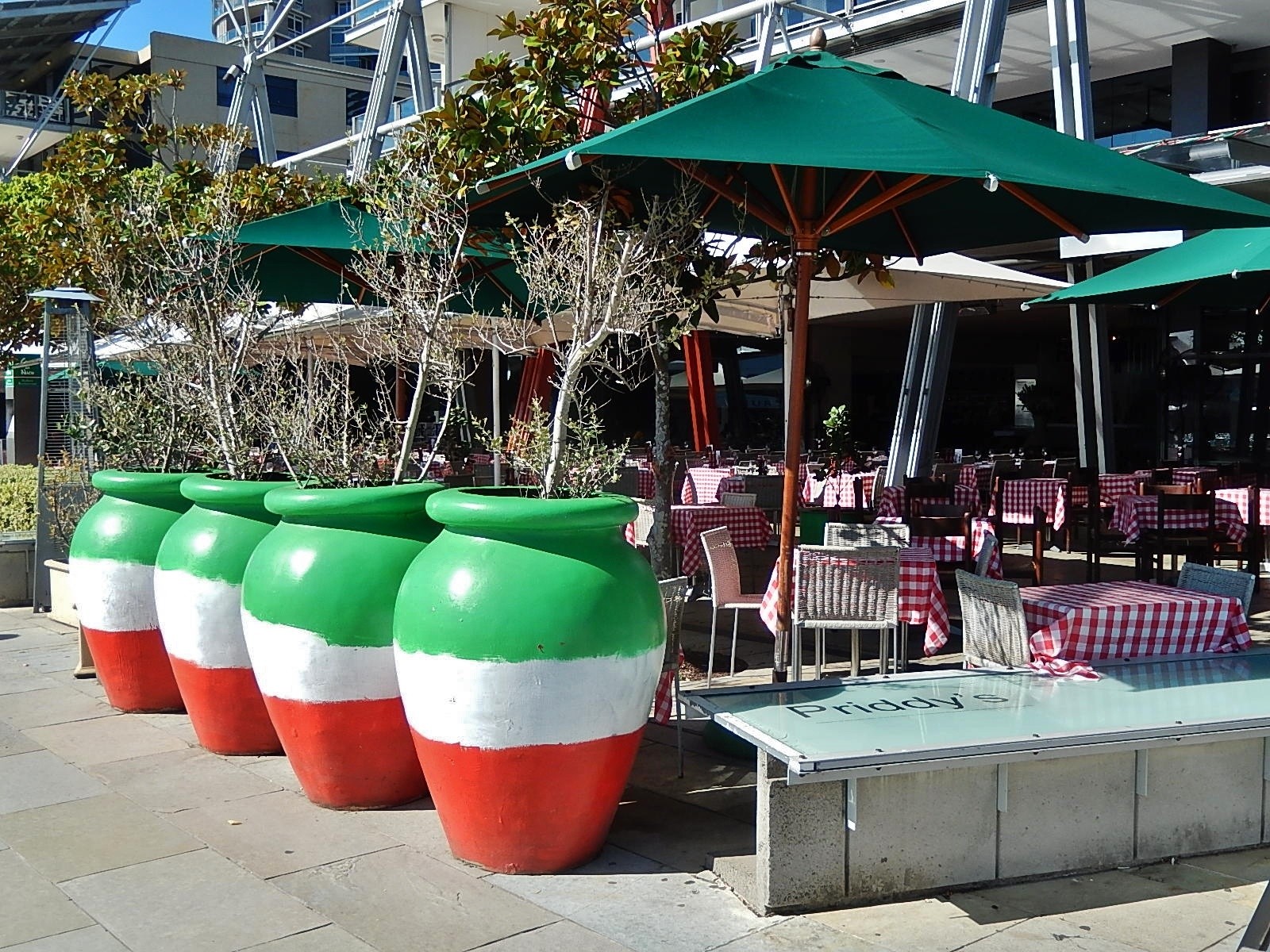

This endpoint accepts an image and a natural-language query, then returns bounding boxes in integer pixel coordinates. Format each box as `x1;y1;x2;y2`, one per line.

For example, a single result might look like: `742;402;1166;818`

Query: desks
1207;486;1270;570
770;460;859;501
953;459;994;486
876;484;1005;580
625;504;775;600
871;516;1005;581
1108;491;1246;575
758;546;949;675
991;477;1099;554
803;469;882;511
680;463;784;507
1052;473;1153;556
429;454;534;488
1132;468;1222;499
1017;580;1253;677
625;456;658;501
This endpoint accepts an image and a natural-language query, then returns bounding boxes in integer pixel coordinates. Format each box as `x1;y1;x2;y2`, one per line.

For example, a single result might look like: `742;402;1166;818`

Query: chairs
387;421;1270;682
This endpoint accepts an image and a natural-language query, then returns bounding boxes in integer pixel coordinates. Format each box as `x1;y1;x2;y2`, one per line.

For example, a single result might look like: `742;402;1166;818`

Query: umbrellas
443;28;1270;682
1025;226;1270;309
14;197;1073;432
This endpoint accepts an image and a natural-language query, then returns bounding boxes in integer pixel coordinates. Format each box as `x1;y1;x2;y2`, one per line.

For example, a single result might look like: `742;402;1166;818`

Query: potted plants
44;115;730;875
